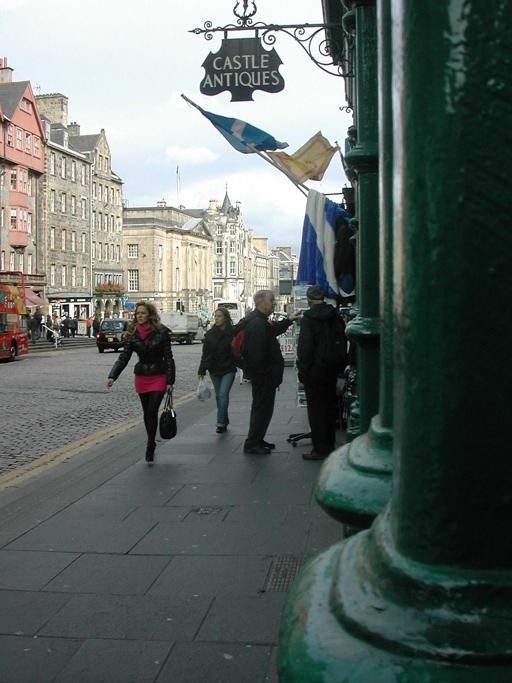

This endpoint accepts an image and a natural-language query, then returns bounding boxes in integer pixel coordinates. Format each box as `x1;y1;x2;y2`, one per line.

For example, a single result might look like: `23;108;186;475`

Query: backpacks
228;318;250;369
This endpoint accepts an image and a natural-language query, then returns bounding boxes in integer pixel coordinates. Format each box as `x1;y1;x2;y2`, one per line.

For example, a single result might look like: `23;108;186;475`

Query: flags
266;129;340;186
204;108;290;154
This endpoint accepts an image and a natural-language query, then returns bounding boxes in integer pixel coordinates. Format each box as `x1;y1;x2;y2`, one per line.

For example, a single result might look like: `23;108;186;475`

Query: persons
106;301;177;464
239;288;303;455
195;306;238;434
25;307;102;347
294;284;348;462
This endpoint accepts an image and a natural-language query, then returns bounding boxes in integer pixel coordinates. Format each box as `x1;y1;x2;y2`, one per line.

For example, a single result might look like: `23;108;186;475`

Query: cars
96;318;132;353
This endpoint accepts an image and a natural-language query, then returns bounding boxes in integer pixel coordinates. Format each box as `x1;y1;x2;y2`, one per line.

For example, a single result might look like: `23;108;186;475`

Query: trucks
160;310;211;345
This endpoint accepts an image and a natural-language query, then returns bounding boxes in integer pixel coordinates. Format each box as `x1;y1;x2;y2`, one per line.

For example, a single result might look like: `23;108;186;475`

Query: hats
307;285;324;299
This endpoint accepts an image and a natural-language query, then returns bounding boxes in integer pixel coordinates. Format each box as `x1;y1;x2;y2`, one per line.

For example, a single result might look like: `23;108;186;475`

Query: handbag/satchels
159;390;177;440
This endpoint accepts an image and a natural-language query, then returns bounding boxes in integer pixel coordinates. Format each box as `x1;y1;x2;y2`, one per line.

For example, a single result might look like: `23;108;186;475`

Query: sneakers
244;439;271;455
303;451;327;460
259;439;275;448
216;419;229;433
